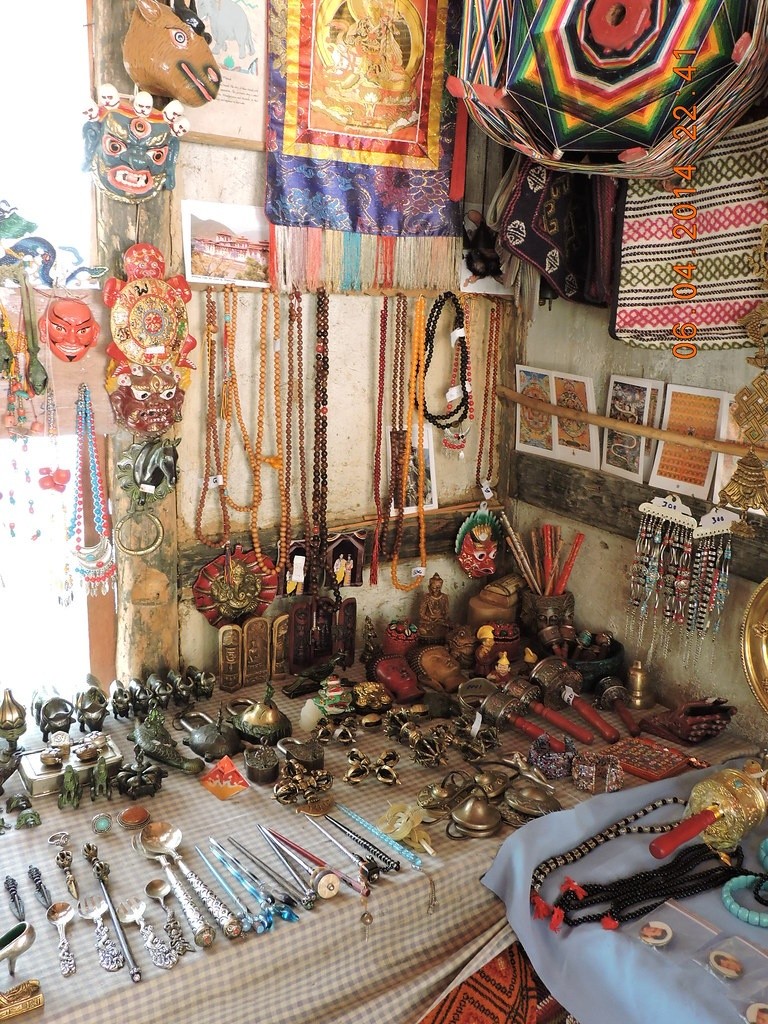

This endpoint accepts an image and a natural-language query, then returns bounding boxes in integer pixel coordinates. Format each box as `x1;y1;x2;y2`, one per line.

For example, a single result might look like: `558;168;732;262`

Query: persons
333;553;347;586
474;625;499;677
639;921;668;940
522;648;540;677
714;953;744;976
343;554;354;586
417;573;451;637
485;651;516;691
749;1007;768;1024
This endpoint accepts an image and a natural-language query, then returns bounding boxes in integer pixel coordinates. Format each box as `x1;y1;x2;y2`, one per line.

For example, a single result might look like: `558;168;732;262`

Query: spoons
140;821;243;939
46;902;76;978
131;833;216;948
145;879;197;956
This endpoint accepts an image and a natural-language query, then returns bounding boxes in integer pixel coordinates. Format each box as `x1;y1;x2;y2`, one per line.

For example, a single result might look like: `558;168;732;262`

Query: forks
77;895;126;972
116;897;179;969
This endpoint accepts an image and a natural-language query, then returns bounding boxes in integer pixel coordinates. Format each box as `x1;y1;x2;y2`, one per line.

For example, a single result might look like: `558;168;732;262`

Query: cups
520;590;575;642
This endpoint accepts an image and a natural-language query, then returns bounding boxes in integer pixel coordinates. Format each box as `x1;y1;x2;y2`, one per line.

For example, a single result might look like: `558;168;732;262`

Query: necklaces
0;249;510;603
526;797;768;929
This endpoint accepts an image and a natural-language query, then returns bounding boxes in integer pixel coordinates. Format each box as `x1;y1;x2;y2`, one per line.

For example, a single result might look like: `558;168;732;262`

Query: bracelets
758;837;768;873
723;874;768;929
